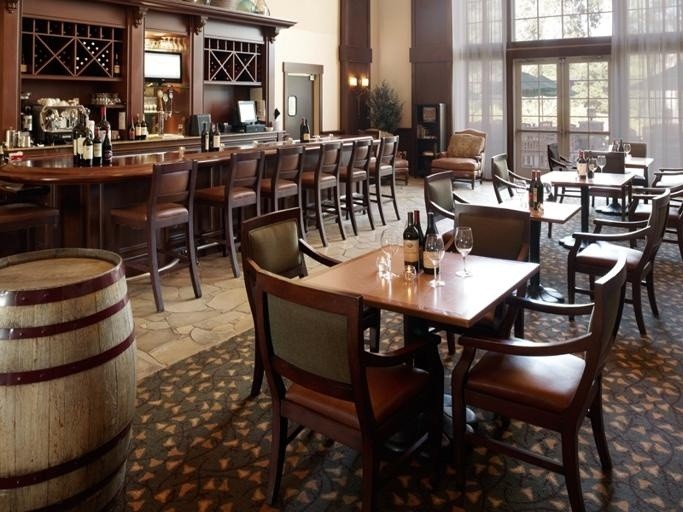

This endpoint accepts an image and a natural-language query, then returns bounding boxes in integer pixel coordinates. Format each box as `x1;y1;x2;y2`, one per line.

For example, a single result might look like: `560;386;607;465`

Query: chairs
583;150;625;206
567;187;671;329
246;259;444;509
1;168;62;250
455;256;628;506
624;143;648;206
490;152;534;204
633;168;683;262
547;143;576;204
428;170;471;248
428;202;533;329
238;206;343;401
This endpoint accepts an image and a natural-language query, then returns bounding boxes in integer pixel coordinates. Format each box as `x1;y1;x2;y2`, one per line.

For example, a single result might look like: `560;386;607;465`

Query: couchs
432;128;487;189
374;129;409;185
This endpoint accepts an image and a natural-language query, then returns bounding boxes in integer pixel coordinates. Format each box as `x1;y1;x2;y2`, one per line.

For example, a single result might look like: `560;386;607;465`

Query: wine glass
623;143;631;163
541;182;551;212
424;233;445;288
380;230;399;280
453;226;474;277
589;155;605;181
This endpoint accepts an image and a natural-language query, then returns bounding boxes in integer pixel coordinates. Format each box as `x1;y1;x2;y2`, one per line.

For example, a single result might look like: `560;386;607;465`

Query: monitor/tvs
144;51;182;83
238;100;258;123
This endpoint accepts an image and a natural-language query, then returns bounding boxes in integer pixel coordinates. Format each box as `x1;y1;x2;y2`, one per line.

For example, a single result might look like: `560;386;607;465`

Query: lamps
348;76;370;95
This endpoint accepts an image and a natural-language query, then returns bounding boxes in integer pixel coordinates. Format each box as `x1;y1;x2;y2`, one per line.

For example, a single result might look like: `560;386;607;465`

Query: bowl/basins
264;127;273;132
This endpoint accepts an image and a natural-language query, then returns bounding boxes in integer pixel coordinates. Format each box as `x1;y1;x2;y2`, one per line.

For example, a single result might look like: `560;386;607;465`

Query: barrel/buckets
1;246;139;512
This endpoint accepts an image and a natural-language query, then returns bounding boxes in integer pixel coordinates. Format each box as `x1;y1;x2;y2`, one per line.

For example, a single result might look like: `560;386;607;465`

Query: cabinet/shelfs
191;2;296;134
414;102;447;179
0;2;140;139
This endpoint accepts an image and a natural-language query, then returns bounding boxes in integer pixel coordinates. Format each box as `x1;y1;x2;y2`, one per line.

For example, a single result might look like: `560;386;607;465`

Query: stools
367;135;401;226
254;145;308;246
326;138;376;237
194;149;265;278
300;141;346;247
109;158;202;313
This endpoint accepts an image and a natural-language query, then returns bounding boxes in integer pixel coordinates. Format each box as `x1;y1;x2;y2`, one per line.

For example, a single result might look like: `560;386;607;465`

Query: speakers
191;113;212;136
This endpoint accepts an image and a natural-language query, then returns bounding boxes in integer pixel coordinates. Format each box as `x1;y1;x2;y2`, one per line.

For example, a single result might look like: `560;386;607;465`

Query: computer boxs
239;124;265;133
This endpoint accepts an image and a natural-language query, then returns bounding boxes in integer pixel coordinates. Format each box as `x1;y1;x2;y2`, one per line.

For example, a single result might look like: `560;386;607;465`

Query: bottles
20;95;33;132
205;52;262;82
128;113;147;140
402;210;440;275
612;138;623;152
202;121;219;153
72;106;111;168
529;171;543;209
21;27;121;78
299;118;310;143
576;150;593;179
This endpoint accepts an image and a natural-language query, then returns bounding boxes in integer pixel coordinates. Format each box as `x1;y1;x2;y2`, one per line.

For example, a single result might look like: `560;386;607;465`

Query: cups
95;93;119;106
401;265;416;281
5;129;30;147
177;124;183;135
375;229;387;264
